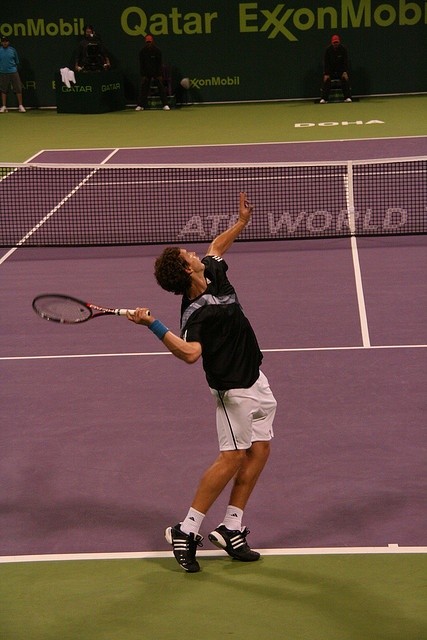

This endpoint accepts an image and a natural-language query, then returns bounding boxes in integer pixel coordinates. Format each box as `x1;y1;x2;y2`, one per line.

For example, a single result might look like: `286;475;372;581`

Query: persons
0;36;26;113
319;35;352;104
134;35;170;111
75;24;111;71
127;191;278;573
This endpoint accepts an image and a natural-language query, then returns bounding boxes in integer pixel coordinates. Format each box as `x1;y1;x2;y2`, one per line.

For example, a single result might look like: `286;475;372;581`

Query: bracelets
147;319;168;340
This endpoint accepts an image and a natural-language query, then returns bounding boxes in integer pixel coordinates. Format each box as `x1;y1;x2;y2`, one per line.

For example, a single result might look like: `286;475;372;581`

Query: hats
0;34;7;41
145;35;152;42
331;35;340;43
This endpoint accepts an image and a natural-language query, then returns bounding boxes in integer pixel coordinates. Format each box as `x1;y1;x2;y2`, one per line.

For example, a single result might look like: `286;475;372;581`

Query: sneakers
163;523;204;574
208;522;260;562
319;99;328;103
343;97;352;103
0;106;8;112
162;104;170;111
134;105;144;112
18;104;26;113
